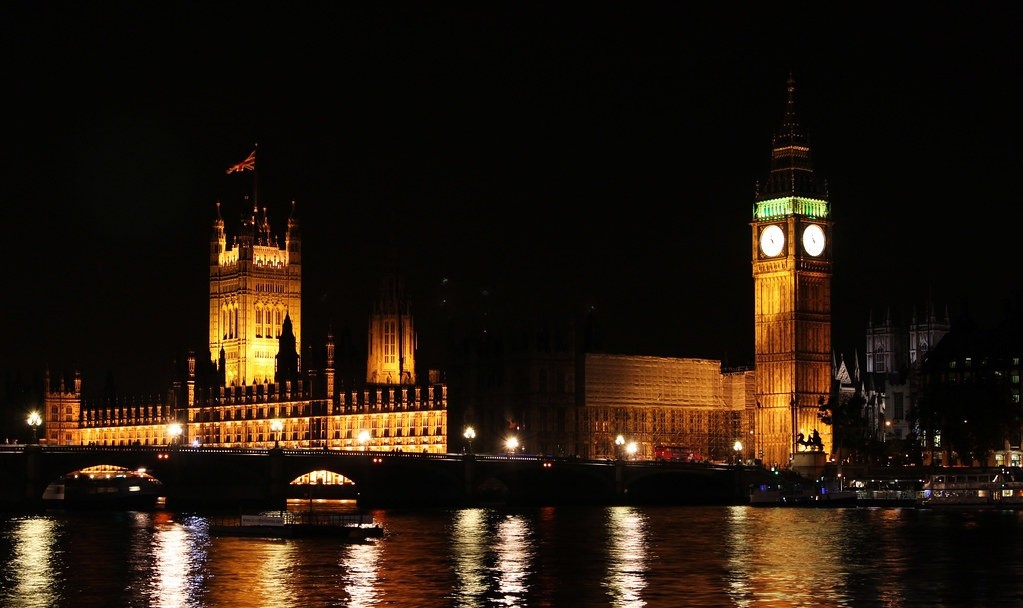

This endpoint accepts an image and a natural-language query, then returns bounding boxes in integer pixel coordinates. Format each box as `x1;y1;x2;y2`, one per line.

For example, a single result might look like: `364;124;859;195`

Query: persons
88;441;95;451
168;442;180;451
727;461;754;470
811;429;819;443
200;444;203;452
536;449;544;462
934;492;978;497
4;439;18;452
391;448;403;457
690;457;713;468
423;449;427;458
133;440;140;452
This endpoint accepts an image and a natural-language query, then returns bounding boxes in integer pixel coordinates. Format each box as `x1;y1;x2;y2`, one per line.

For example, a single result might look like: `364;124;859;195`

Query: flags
227;152;255;175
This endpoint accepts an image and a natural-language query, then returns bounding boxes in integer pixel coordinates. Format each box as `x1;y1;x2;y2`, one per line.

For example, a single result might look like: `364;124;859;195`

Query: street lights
26;410;42;444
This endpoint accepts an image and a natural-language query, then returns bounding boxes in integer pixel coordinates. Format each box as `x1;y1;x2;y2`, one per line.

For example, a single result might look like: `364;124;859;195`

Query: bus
652;444;704;463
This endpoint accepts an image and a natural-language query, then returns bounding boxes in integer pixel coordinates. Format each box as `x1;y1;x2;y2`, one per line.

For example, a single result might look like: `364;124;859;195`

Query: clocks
803;224;826;256
758;225;784;257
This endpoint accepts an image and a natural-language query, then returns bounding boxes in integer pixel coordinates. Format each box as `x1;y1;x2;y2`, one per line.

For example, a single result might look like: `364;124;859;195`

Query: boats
63;476;160;510
748;471;1023;508
206;507;385;541
